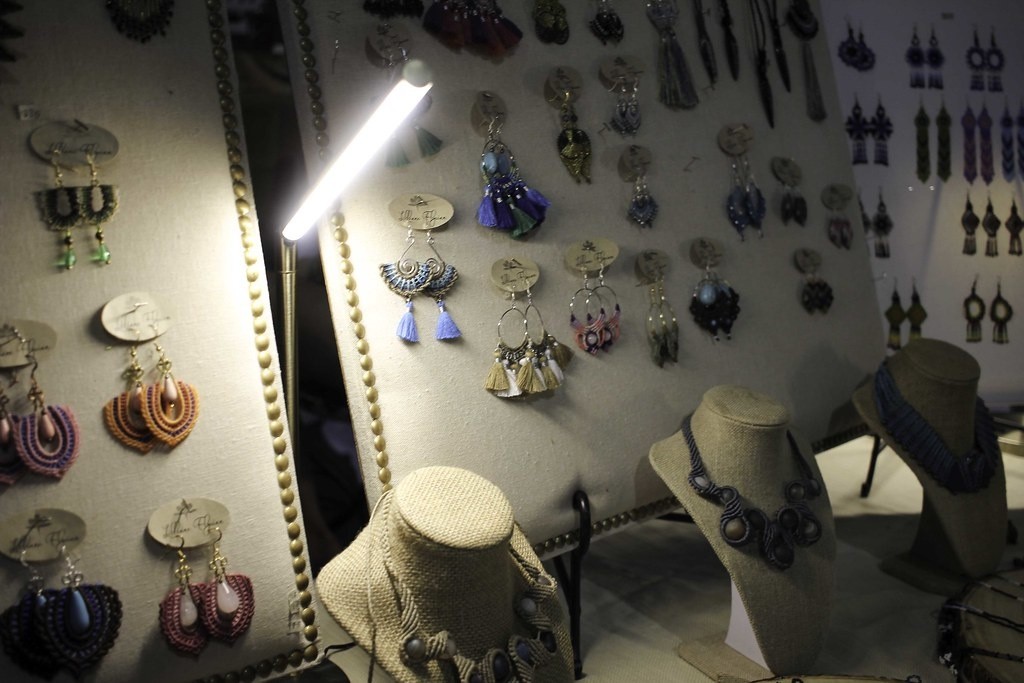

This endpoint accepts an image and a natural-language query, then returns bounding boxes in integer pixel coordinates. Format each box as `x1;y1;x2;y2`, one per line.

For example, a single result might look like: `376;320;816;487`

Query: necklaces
683;411;820;570
366;488;557;683
873;357;1000;495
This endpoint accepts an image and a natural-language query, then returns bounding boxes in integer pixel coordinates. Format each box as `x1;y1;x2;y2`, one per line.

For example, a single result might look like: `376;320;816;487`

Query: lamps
277;58;435;443
